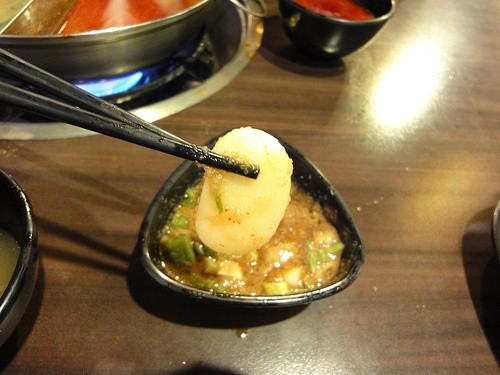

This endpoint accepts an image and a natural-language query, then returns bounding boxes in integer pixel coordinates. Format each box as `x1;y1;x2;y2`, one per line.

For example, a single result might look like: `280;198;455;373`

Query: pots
0;0;218;78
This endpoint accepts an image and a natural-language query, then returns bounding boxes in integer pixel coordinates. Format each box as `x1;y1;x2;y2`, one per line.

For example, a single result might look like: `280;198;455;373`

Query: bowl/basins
139;126;365;310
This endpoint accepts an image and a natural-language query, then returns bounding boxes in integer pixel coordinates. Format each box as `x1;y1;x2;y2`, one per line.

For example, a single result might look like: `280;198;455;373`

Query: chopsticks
0;46;260;181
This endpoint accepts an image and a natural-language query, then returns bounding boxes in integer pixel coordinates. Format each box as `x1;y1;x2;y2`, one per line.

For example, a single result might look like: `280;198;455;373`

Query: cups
0;168;38;323
278;0;397;62
